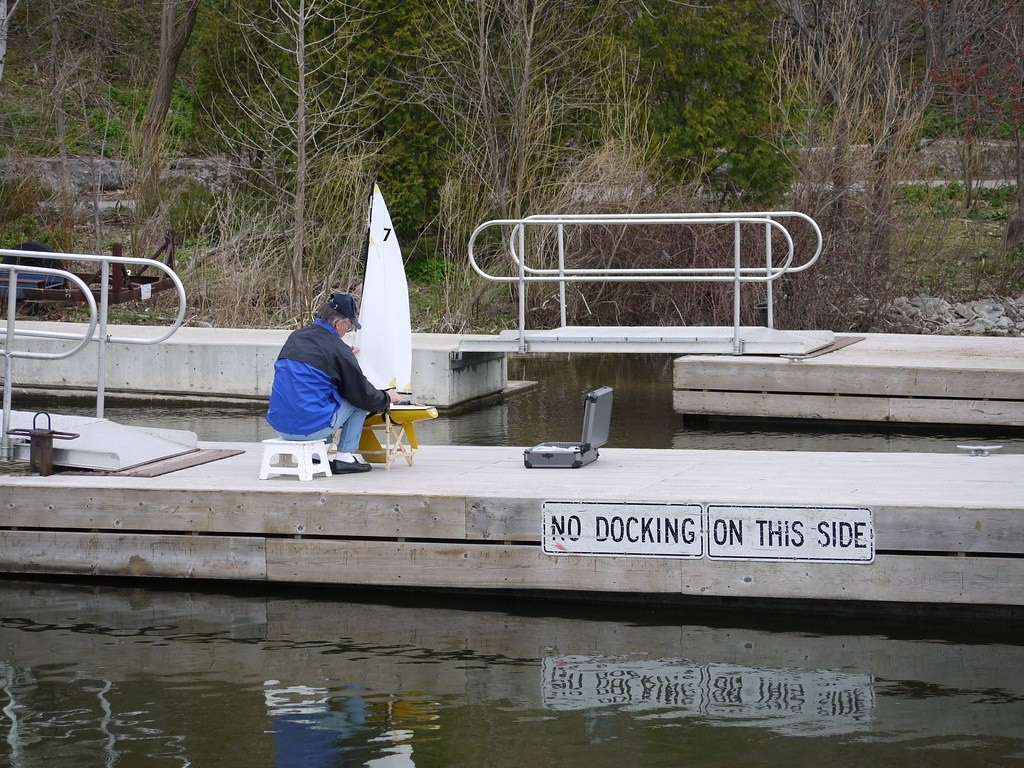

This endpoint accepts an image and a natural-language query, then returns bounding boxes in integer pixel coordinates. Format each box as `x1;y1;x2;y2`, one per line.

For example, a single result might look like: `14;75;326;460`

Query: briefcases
523;384;614;468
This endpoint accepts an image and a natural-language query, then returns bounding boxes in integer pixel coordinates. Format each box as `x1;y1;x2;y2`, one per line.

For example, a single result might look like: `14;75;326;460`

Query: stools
258;438;334;481
263;677;333;718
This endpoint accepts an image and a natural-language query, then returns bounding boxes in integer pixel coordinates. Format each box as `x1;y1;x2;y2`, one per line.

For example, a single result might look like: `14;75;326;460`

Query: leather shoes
332;456;372;474
312;458;333;470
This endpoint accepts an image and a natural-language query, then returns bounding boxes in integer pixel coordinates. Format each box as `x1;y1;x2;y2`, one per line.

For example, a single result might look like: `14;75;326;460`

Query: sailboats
320;180;439;449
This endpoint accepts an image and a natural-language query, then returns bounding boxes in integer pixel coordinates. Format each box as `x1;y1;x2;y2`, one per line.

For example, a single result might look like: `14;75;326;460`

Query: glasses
335;320;354;332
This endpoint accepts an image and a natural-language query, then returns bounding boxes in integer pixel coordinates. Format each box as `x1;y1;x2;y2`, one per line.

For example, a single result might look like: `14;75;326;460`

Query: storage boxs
523;386;614;470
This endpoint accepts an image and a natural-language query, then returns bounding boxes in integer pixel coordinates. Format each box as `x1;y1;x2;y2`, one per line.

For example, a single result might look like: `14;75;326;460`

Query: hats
326;292;361;329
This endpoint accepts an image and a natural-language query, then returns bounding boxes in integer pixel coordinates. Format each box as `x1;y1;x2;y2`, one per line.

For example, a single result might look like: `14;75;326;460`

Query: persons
264;293;401;475
